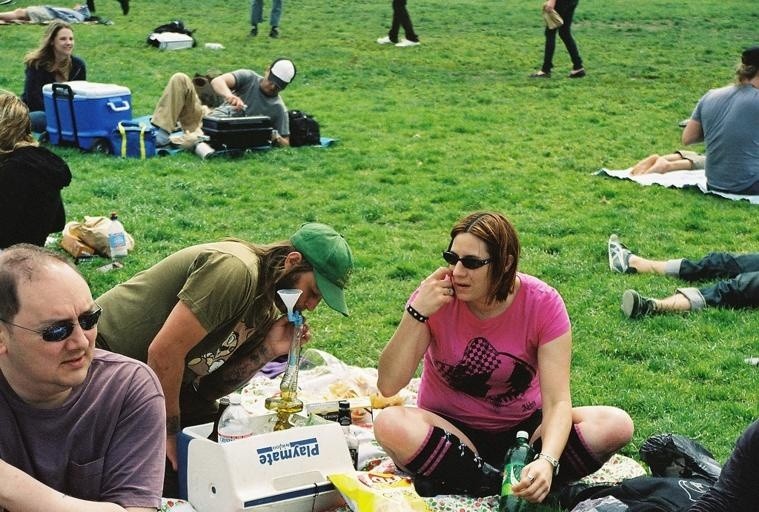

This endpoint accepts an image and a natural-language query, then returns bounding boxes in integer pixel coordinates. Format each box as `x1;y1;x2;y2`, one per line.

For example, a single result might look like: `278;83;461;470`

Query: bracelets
404;303;429;324
533;453;562;477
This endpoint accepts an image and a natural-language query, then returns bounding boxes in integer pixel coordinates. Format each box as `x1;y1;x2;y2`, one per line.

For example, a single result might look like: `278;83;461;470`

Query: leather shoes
567;70;585;78
413;474;447;497
549;479;589;508
528;72;550;78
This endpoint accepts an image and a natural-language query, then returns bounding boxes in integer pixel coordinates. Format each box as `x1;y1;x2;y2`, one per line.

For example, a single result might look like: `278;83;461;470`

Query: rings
527;474;535;483
447;287;452;295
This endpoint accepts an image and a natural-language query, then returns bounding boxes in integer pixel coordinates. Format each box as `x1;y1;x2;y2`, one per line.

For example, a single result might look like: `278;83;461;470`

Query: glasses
1;302;104;342
440;250;491;269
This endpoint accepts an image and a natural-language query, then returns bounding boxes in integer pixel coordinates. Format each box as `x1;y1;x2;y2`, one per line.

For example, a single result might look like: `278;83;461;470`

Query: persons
85;0;130;17
604;227;758;319
629;148;706;177
248;0;283;37
374;0;421;49
92;220;354;500
0;4;91;27
19;18;88;135
147;57;298;160
0;88;75;251
680;47;759;198
0;240;168;512
373;206;635;512
527;0;586;79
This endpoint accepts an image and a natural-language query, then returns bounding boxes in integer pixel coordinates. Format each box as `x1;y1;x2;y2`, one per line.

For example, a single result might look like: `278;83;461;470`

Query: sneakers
394;39;421;46
607;233;637;273
376;36;393;45
270;27;279;37
251;26;258;36
621;289;658;319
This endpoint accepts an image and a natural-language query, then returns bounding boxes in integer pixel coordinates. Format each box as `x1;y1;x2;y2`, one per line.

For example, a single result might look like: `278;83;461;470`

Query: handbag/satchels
289;109;321;147
78;215;134;258
109;119;156;159
147;32;194;50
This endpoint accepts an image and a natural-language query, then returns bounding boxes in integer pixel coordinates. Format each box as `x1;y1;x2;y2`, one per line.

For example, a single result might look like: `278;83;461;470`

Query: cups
195;142;216;159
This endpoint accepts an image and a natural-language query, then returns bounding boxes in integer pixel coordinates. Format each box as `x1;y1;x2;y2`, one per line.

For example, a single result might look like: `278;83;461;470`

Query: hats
268;58;296;90
741;48;758;65
289;221;354;317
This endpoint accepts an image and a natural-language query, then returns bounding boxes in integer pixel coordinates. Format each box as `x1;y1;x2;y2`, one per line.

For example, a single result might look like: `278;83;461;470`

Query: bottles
497;428;540;512
217;395;253;444
107;211;128;260
206;397;233;444
334;399;361;473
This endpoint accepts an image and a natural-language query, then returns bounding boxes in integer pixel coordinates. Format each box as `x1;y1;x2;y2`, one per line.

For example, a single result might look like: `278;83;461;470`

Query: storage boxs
201;109;276;154
178;405;363;512
38;78;135;155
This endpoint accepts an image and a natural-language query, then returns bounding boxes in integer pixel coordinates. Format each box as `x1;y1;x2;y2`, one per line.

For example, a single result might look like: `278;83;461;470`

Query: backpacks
567;476;713;511
